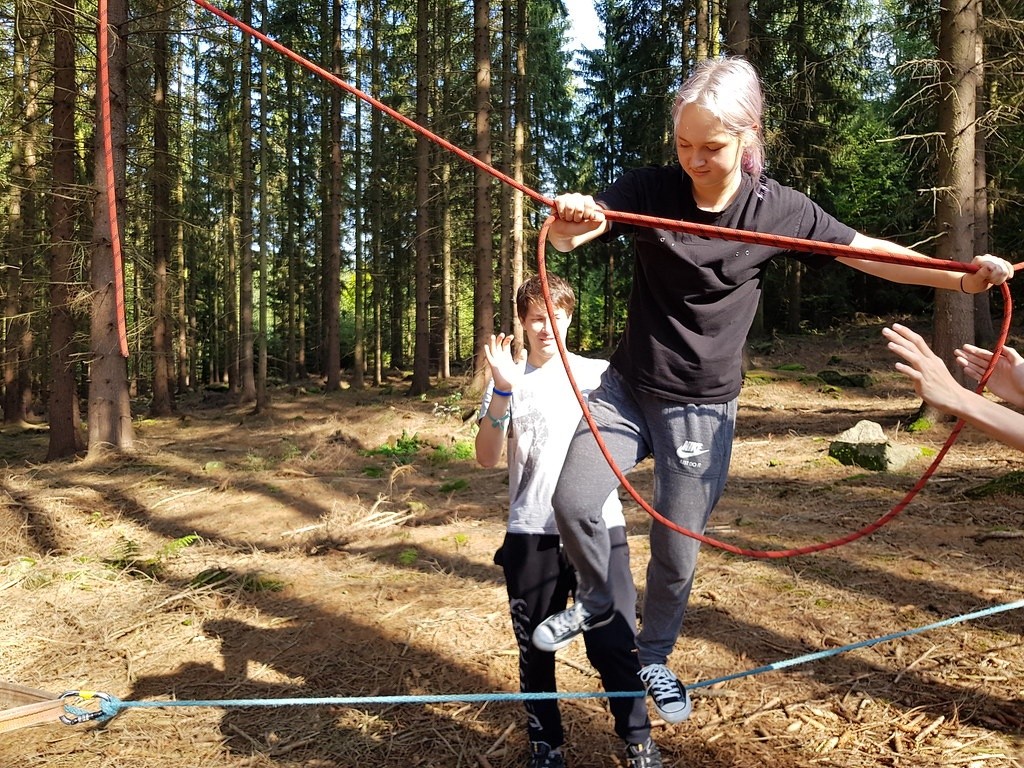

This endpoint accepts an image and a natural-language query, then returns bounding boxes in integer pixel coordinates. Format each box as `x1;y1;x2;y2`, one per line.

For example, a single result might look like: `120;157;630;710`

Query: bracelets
493;387;512;396
486;410;509;430
960;277;971;294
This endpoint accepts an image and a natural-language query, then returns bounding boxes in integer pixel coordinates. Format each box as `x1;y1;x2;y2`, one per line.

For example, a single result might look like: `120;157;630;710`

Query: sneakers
625;734;662;768
527;741;566;768
532;600;615;651
637;663;691;723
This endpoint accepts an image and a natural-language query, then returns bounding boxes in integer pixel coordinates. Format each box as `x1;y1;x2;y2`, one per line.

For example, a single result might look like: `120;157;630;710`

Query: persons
476;275;662;768
882;323;1024;451
533;58;1014;723
